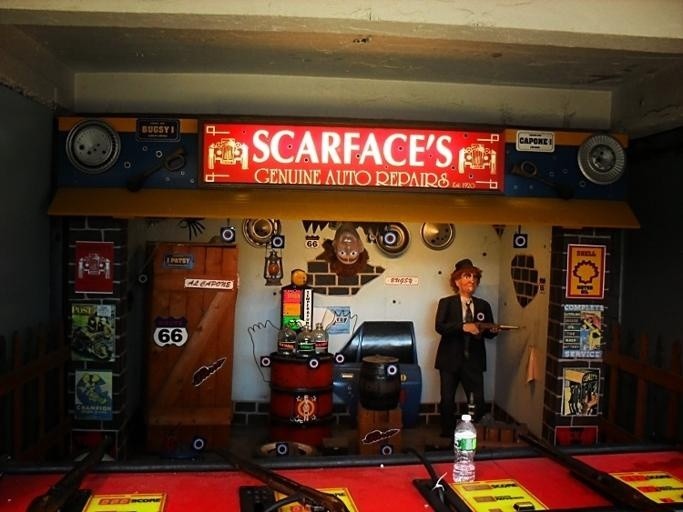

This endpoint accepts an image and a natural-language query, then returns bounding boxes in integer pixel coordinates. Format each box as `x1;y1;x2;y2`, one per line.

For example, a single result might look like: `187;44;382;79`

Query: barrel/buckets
269;351;334;444
358;355;401;411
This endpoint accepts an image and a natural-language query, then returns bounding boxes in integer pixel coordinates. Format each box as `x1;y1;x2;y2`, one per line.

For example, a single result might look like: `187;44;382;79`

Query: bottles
452;414;477;483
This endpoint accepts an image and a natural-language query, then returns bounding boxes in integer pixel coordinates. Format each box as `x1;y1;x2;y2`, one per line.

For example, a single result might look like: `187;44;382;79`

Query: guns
516;434;658;508
27;442;104;511
212;448;349;512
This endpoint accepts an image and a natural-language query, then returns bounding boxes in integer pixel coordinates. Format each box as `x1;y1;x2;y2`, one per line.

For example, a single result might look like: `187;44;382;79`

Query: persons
321;221;376;277
435;259;501;431
